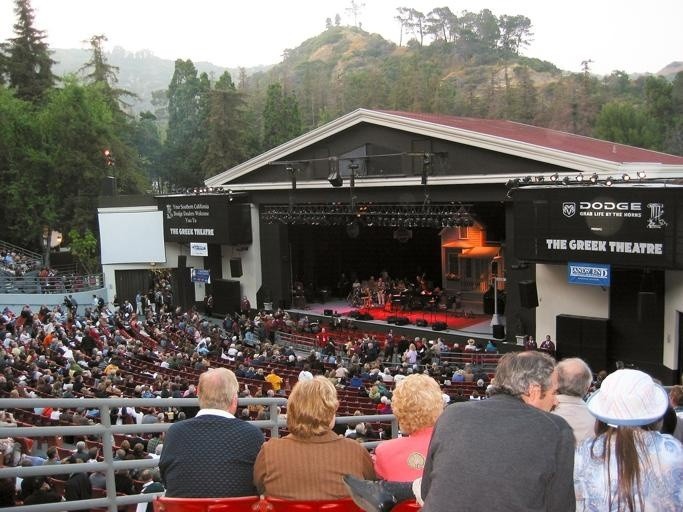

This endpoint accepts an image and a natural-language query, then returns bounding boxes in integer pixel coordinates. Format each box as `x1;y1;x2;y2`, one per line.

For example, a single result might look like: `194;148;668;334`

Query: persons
184;295;555;411
338;273;448;312
1;247;187;512
163;351;682;512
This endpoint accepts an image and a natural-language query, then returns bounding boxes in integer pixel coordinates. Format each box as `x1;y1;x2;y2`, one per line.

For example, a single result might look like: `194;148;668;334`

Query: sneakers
343;474;397;511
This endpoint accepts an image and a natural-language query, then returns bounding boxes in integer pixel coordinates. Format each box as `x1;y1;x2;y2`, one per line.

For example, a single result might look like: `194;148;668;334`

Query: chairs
0;330;504;511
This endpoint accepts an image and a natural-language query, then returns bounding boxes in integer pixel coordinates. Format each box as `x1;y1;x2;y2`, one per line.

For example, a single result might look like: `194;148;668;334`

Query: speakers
212;280;241;314
324;309;332;316
517;281;539;309
386;315;448;331
492;325;505;339
229;259;242;277
556;314;609;373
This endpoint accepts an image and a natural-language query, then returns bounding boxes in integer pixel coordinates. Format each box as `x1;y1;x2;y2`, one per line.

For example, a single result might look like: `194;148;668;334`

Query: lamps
175;185;233;202
504;169;646;186
264;206;473;227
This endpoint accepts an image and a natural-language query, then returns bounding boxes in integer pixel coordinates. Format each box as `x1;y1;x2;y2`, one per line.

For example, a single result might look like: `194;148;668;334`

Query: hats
586;368;666;426
